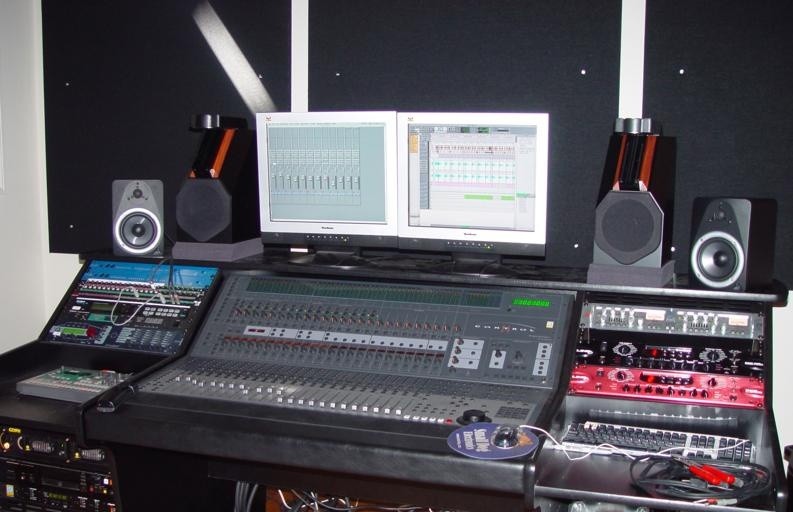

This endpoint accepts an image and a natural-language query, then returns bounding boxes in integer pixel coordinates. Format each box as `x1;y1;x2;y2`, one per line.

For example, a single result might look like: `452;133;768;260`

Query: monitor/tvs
397;112;552;276
255;110;396;269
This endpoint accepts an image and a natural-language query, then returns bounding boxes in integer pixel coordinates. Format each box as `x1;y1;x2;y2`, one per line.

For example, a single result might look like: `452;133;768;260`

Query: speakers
111;179;167;259
687;196;778;291
587;133;677;287
170;126;264;262
